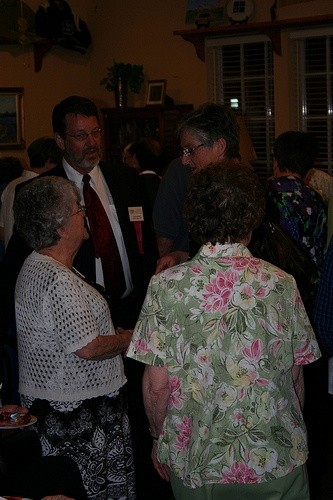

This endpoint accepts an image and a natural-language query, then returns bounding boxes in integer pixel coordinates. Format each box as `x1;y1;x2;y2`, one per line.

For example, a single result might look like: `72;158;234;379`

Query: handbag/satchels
253;222;317;285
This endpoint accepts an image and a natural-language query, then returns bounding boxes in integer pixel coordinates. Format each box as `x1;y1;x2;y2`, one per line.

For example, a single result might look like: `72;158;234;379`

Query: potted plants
100;60;144;112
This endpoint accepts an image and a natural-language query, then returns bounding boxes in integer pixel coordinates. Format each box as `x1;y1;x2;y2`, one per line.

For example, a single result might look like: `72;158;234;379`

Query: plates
0;412;37;429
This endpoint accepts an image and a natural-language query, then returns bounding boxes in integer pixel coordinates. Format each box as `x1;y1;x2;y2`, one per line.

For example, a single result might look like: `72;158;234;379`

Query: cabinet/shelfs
99;103;193;168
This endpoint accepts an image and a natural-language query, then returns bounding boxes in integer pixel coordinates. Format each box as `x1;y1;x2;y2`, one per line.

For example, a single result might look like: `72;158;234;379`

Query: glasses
70;204;86;218
183;142;205;156
66;127;104;141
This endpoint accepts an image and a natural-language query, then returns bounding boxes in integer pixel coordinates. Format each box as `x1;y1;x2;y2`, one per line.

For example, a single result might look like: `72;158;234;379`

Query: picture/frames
0;86;27;150
145;79;167;107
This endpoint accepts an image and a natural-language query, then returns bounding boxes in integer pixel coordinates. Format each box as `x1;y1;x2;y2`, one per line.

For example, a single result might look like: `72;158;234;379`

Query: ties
82;173;127;302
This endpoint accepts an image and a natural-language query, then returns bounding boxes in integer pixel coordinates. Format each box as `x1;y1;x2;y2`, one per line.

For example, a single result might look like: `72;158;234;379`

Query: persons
269;152;333;255
7;95;140;305
259;130;328;268
155;103;316;310
124;158;323;500
0;136;65;255
98;132;177;264
14;176;133;500
